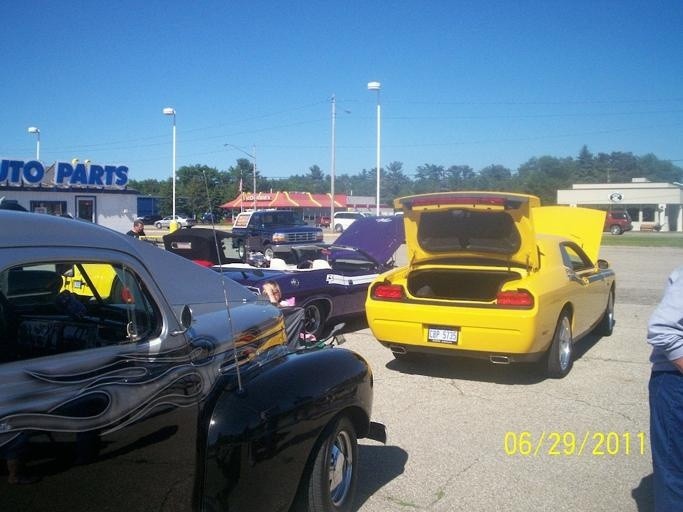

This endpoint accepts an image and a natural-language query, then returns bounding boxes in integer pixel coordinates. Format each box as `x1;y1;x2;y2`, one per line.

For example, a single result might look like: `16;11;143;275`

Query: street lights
28;127;40;162
224;144;256;211
368;81;380;216
331;102;351;233
164;108;176;219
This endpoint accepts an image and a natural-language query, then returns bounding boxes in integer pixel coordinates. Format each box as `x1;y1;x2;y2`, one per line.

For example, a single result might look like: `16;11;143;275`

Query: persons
647;266;683;512
262;280;288;307
126;219;145;238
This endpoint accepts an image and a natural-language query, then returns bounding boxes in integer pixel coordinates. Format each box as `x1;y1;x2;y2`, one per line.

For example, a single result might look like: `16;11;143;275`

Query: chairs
271;259;286;268
314;260;329;268
